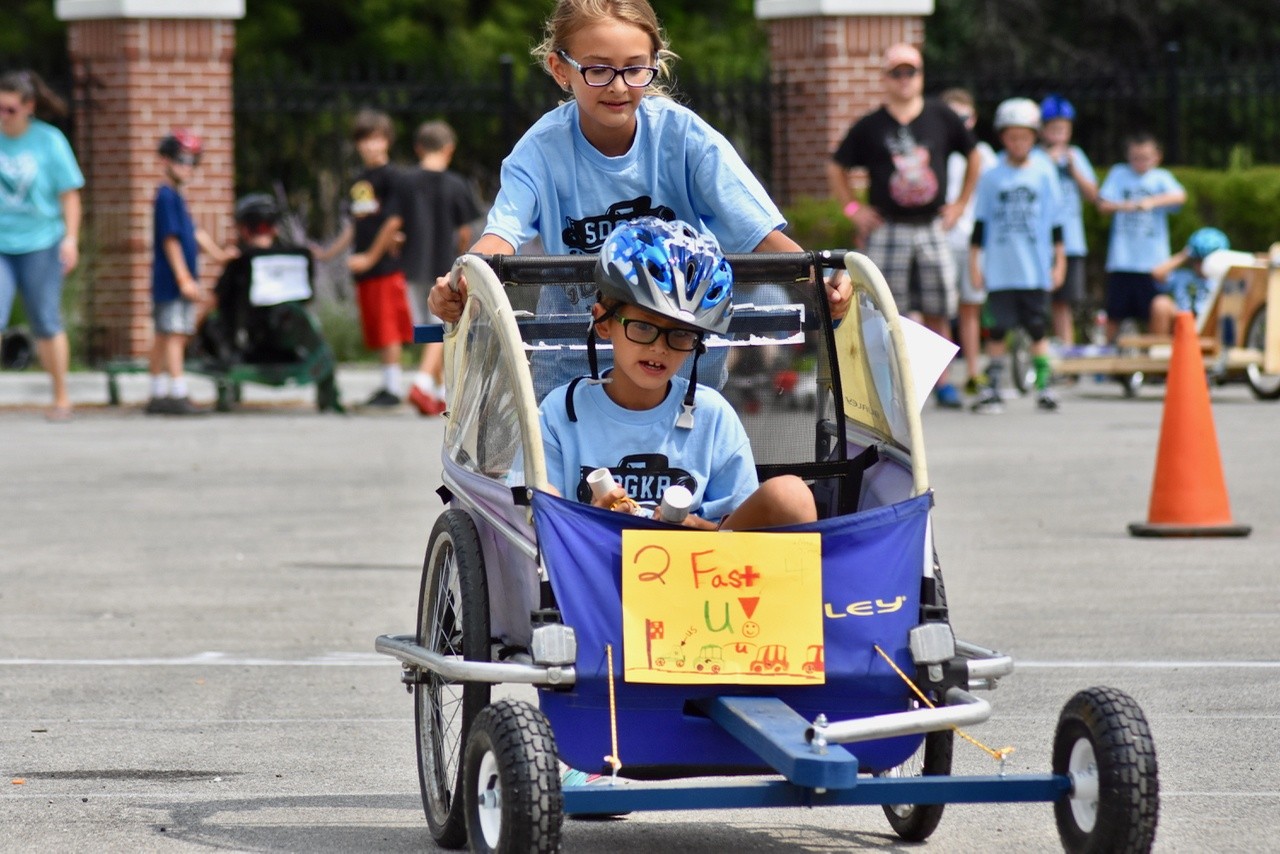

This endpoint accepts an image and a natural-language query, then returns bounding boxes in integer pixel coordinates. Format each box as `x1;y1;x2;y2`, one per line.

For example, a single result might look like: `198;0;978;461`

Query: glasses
555;50;659;88
600;302;705;351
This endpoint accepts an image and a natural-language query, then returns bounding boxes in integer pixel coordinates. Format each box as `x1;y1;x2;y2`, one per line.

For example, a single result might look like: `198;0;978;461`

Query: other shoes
45;399;74;421
144;389;400;414
407;386;448;419
936;370;1058;416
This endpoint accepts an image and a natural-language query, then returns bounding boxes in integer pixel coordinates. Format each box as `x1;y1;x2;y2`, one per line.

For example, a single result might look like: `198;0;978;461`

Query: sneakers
563;767;635;813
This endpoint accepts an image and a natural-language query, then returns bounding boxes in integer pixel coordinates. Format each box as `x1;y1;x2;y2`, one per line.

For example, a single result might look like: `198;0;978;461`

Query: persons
144;132;242;415
195;195;349;415
428;0;852;816
725;34;1243;418
401;124;483;416
0;72;84;422
304;112;411;408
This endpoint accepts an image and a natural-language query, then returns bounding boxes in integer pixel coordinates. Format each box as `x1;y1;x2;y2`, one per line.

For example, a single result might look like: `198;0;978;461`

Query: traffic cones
1124;310;1254;540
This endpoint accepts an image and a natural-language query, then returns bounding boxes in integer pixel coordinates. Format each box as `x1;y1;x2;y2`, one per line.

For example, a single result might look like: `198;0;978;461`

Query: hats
1042;96;1072;121
882;44;924;74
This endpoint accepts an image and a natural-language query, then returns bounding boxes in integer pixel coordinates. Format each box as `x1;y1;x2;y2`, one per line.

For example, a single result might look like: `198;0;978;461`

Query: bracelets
843;201;860;220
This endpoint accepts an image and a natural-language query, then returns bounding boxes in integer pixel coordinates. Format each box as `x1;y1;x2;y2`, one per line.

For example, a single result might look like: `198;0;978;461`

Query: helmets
235;193;275;226
157;131;202;166
994;99;1040;132
594;215;733;336
